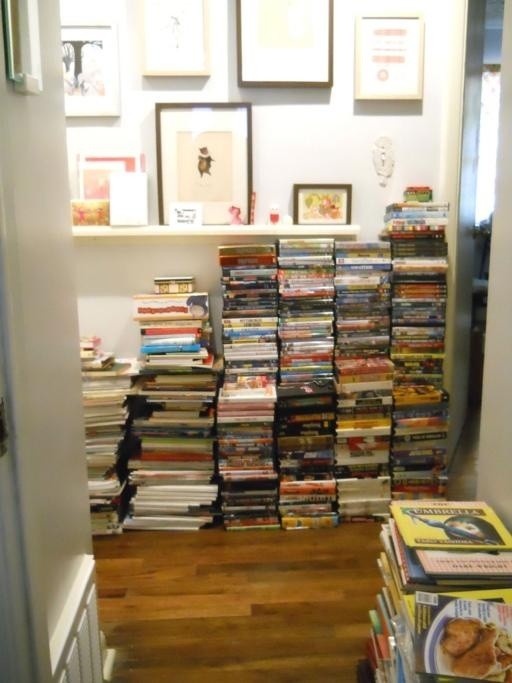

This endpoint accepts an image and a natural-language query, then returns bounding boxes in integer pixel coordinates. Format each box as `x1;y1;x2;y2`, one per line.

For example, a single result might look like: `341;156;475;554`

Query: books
78;203;450;539
365;501;512;682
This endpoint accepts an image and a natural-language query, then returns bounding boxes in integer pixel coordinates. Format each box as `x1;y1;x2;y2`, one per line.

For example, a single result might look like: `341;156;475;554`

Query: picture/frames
153;102;255;224
1;0;25;82
136;0;212;79
234;0;335;86
349;12;426;102
291;182;351;224
61;24;119;118
